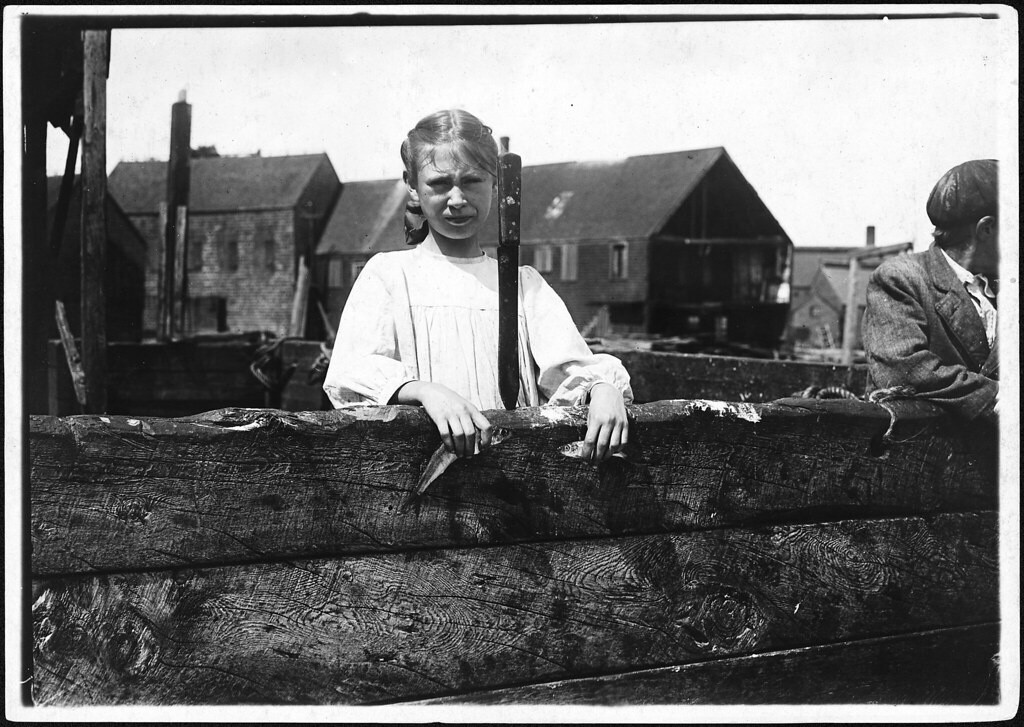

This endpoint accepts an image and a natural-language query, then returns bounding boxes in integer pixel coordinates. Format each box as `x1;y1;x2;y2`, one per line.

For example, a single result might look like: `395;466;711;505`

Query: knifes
496;154;522;411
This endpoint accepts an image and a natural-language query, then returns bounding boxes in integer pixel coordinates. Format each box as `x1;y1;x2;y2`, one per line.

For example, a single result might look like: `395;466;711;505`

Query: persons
325;109;635;460
859;158;998;423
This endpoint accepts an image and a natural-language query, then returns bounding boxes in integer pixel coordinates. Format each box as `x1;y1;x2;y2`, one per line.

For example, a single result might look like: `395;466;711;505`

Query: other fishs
409;426;517;507
557;440;628;459
